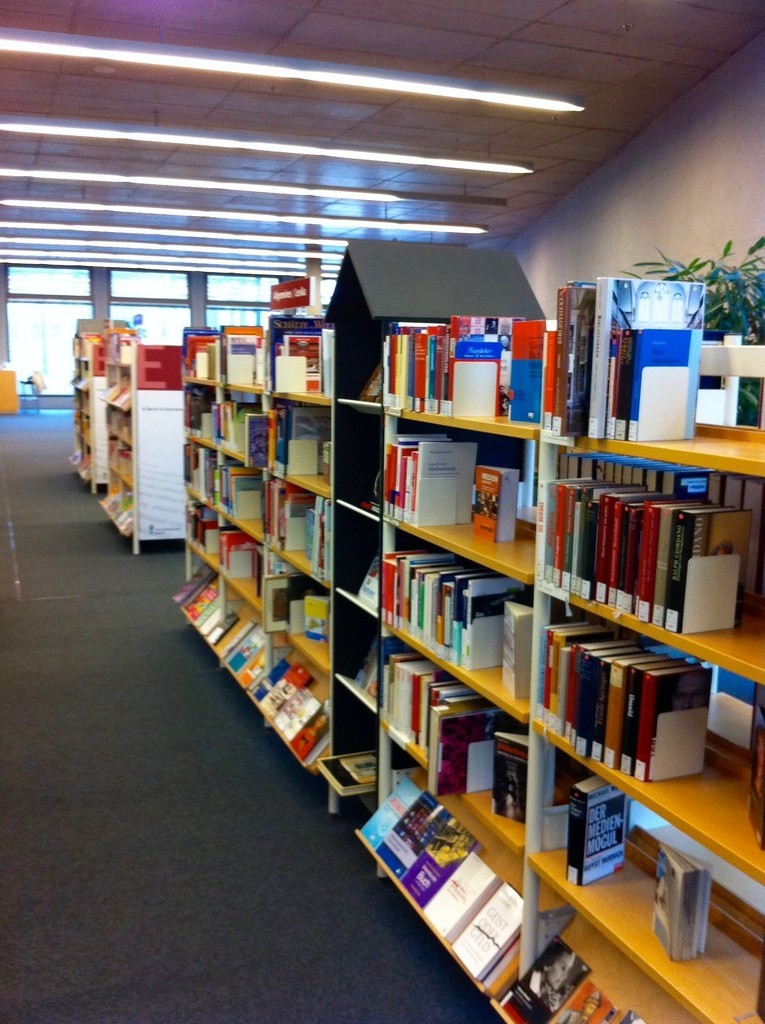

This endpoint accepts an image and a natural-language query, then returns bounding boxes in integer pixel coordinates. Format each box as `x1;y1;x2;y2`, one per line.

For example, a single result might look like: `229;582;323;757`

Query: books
381;638;533;823
557;278;712;441
62;330;142;533
386;314;561;435
746;683;765;850
176;318;334;400
652;840;715;960
175;564;331;765
565;771;629;887
381;548;533;703
170;509;332;665
182;451;332;572
538;620;712;783
543;455;764;640
358;775;526;987
382;429;520;549
185;387;329;487
500;934;647;1024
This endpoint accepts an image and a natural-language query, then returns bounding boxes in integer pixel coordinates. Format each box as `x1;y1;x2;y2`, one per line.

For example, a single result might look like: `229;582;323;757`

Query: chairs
21;369;39;415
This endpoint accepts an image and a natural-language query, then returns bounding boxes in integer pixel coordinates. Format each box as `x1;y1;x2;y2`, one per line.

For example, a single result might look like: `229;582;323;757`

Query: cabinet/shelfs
73;327;764;1024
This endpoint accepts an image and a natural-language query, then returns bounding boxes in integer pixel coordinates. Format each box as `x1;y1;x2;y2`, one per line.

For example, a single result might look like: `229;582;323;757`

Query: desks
0;369;18;415
